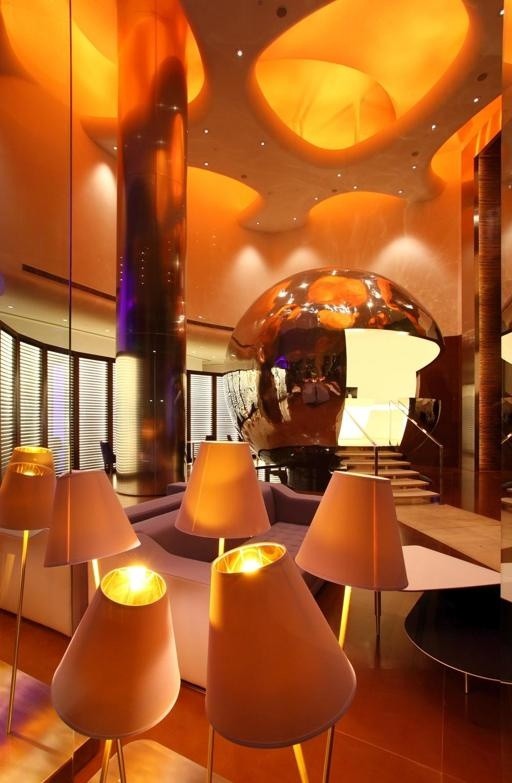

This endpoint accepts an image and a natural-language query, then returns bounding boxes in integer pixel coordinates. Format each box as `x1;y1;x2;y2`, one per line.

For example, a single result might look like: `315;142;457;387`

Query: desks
374;543;511;697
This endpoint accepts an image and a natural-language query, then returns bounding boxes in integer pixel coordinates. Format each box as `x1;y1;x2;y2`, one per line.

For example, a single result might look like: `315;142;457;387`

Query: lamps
52;564;181;762
1;445;57;580
177;443;272;604
203;540;359;779
296;467;407;624
43;468;142;591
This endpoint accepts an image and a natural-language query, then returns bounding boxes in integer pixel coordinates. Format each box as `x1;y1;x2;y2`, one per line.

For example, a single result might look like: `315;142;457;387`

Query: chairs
227;434;258;467
